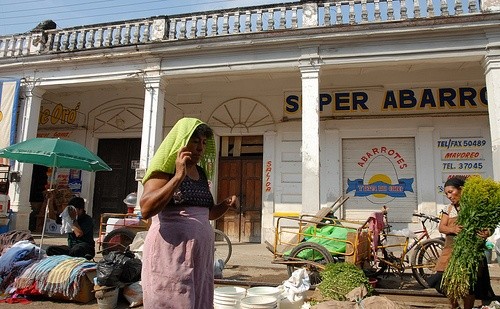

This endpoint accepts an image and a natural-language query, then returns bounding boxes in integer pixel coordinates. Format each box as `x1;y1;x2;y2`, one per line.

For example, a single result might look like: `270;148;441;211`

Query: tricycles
272;210;447;290
96;212;232;268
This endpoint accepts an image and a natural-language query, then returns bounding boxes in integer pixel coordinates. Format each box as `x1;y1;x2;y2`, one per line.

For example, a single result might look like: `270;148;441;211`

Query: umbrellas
0;136;113;261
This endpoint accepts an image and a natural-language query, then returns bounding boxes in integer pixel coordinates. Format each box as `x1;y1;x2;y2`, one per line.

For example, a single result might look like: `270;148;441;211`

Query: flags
0;80;20;165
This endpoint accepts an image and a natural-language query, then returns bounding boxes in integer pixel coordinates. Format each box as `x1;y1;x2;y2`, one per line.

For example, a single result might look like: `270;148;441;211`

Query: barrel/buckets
214;287;246;309
247;287;281;309
241;296;277;309
96;289;118;309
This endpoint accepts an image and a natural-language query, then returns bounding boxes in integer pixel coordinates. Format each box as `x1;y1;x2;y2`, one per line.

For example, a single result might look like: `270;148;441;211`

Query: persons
28;164;48;232
46;197;96;260
432;178;490;309
139;117;239;309
